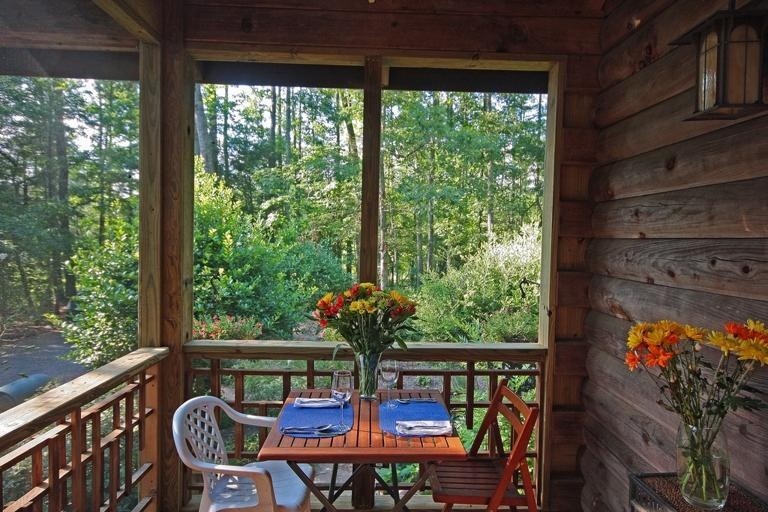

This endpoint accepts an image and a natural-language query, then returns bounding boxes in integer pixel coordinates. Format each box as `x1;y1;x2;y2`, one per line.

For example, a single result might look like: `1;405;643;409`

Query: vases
353;352;383;399
674;422;731;510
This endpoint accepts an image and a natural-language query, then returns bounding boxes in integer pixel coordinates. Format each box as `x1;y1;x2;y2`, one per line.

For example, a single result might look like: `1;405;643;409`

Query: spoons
397;421;445;430
280;425;332;432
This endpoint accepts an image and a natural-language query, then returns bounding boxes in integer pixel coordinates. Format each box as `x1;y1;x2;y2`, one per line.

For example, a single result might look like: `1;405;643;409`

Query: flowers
304;283;421;399
623;319;767;500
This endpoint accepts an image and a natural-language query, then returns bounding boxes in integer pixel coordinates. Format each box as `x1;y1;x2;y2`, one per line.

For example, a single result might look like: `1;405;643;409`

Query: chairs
173;392;315;512
388;378;539;511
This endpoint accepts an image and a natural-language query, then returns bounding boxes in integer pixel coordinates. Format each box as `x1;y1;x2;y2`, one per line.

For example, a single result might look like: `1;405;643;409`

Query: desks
255;386;473;511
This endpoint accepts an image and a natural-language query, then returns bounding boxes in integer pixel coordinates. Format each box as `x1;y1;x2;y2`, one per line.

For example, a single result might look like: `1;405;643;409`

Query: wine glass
332;369;354;432
377;359;399;410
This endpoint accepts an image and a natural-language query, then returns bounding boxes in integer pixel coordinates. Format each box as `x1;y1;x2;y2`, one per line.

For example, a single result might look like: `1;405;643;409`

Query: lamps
665;1;767;122
